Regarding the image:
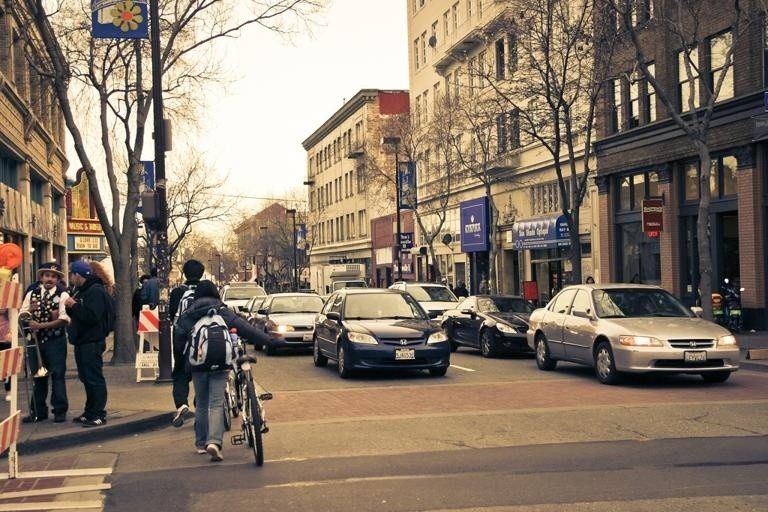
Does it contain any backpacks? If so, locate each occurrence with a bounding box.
[95,287,116,337]
[182,305,240,372]
[172,284,197,327]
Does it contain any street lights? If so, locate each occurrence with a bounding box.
[214,254,221,287]
[283,208,298,287]
[379,134,402,279]
[207,258,213,283]
[257,225,268,285]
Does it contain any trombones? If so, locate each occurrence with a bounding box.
[18,312,53,422]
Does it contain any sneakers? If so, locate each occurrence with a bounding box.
[22,412,48,423]
[197,448,208,454]
[172,404,189,428]
[206,444,223,462]
[72,411,107,427]
[54,413,66,422]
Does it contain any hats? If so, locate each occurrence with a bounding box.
[36,262,65,279]
[68,261,92,278]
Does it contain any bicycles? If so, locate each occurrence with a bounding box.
[222,328,273,469]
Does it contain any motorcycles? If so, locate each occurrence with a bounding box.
[692,284,724,327]
[721,276,747,332]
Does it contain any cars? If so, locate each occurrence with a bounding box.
[519,279,744,389]
[309,284,452,381]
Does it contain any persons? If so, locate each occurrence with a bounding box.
[448,279,469,299]
[169,259,204,428]
[140,268,158,310]
[586,277,594,284]
[132,274,158,330]
[89,262,116,295]
[64,261,116,427]
[18,263,71,423]
[173,279,281,461]
